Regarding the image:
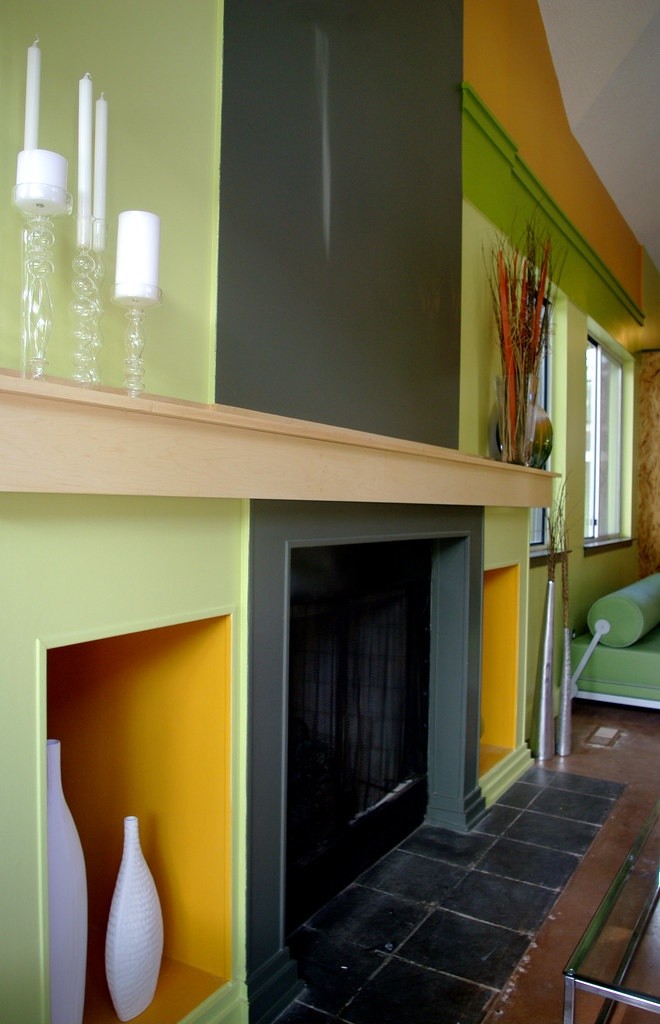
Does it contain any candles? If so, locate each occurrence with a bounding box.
[77,73,94,250]
[93,90,109,250]
[16,148,67,215]
[116,211,160,307]
[24,34,42,152]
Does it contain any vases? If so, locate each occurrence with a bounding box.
[556,627,572,756]
[488,371,553,470]
[105,815,164,1021]
[529,581,555,760]
[46,740,87,1024]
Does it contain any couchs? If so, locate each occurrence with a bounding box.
[570,573,660,709]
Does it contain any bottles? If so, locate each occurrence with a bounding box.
[105,815,163,1021]
[47,739,86,1024]
